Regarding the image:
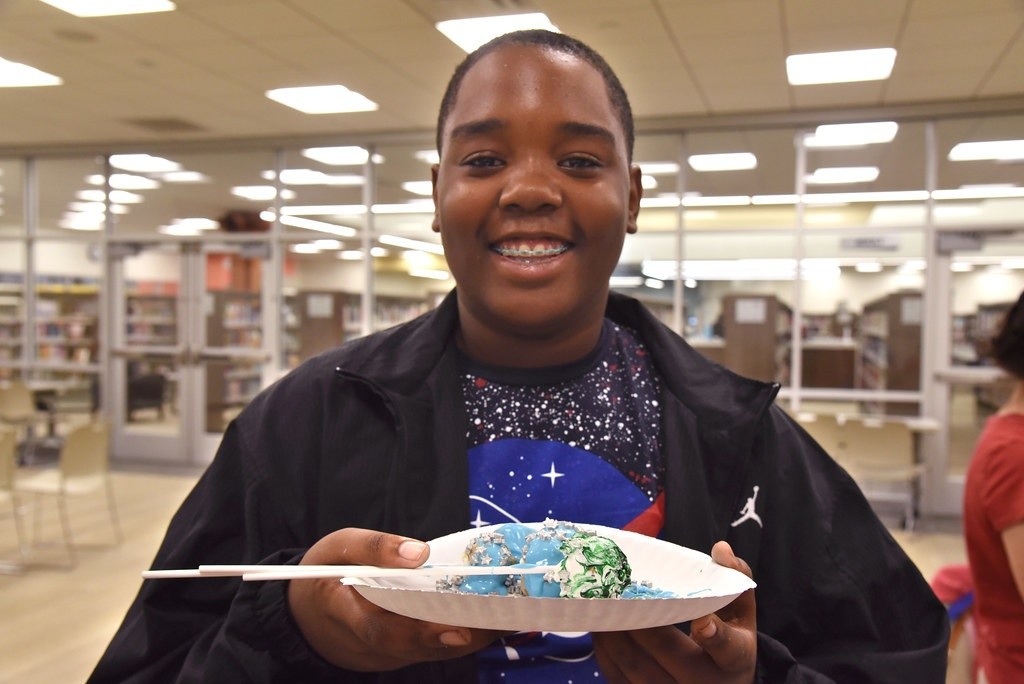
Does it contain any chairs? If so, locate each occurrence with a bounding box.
[0,377,122,575]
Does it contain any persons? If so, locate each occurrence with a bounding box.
[964,289,1023,684]
[86,29,952,684]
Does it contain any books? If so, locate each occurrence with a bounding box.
[0,297,428,432]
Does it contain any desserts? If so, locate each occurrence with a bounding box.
[452,519,634,599]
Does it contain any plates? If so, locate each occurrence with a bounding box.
[348,523,757,631]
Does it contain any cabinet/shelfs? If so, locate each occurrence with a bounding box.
[724,293,792,407]
[970,298,1015,410]
[849,289,927,417]
[0,284,427,449]
